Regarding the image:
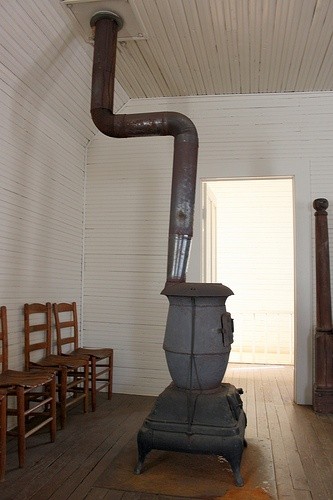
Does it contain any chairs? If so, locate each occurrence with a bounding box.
[0,302,114,482]
